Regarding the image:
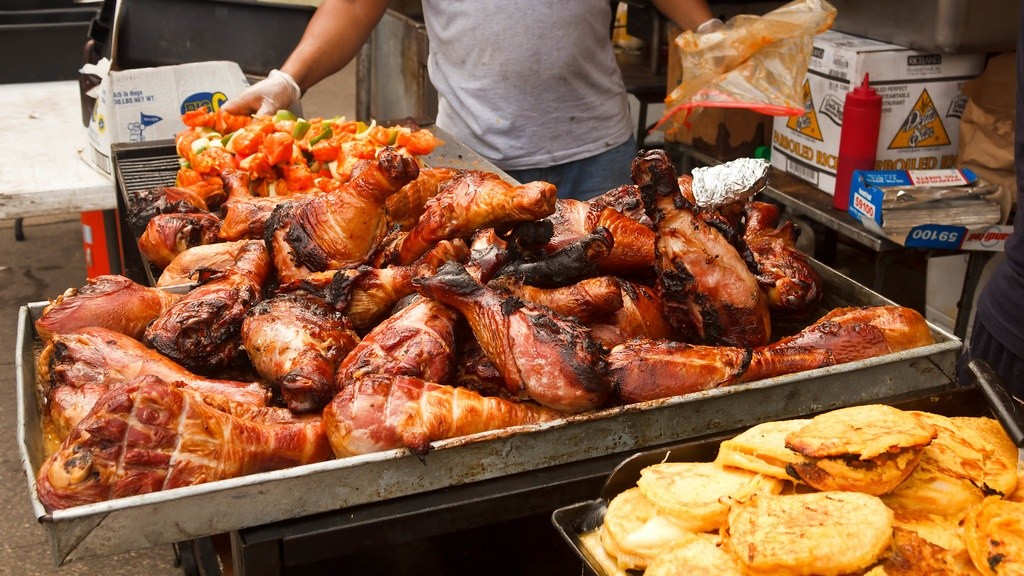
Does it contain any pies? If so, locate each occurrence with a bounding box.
[600,401,1024,576]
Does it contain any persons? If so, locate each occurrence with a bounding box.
[218,1,729,204]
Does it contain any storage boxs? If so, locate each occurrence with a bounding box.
[77,57,250,182]
[769,30,984,199]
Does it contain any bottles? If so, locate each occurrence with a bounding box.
[832,72,884,212]
